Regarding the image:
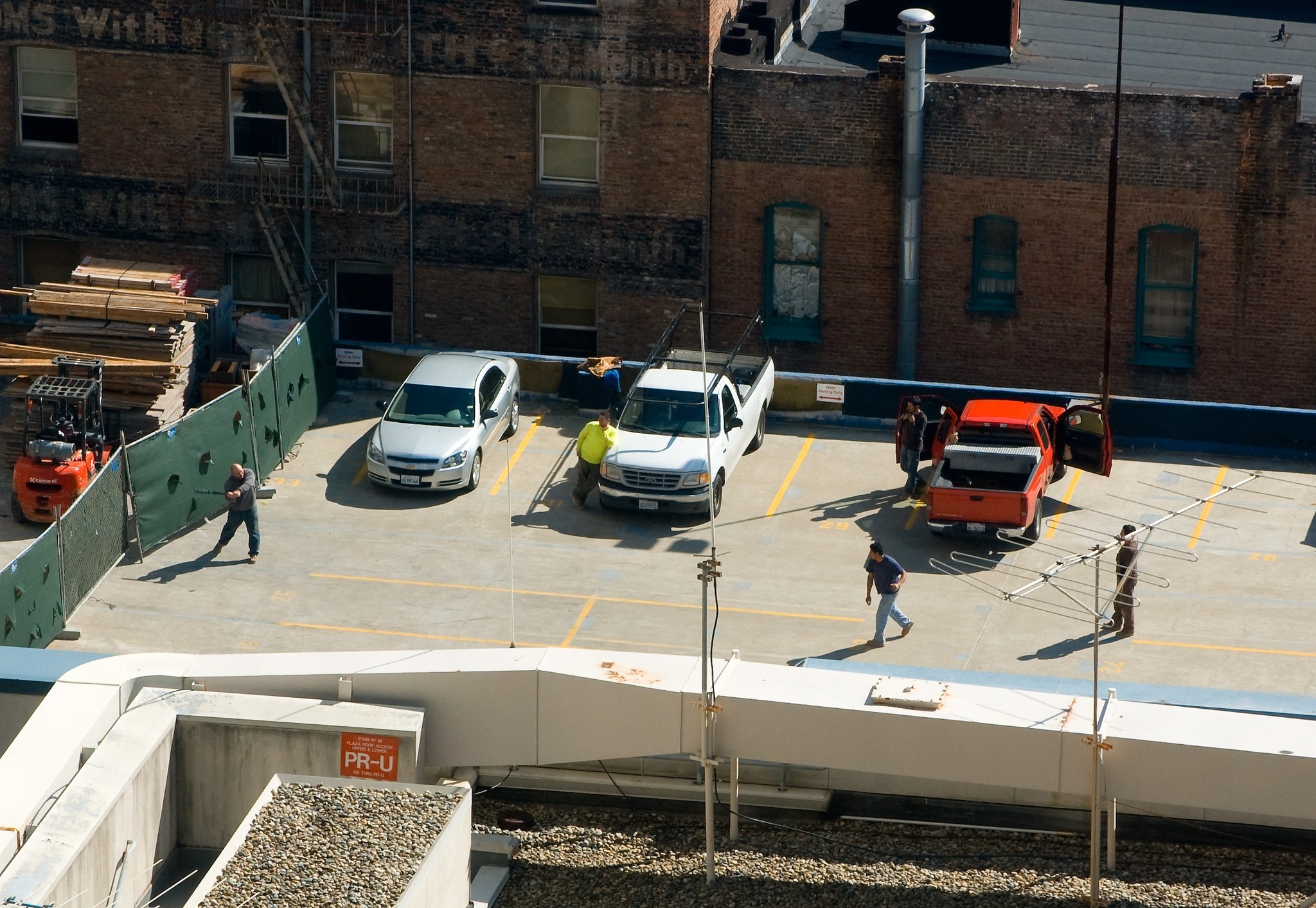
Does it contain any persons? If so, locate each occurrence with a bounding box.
[896,396,927,501]
[213,463,260,563]
[1102,524,1138,637]
[570,411,616,510]
[865,542,914,647]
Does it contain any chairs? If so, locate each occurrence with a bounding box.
[700,393,728,423]
[643,392,672,424]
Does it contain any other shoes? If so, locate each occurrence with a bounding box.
[902,620,914,636]
[915,482,927,491]
[1115,632,1134,638]
[578,504,585,509]
[570,490,582,505]
[1103,623,1121,630]
[867,640,885,647]
[900,489,913,500]
[249,554,258,563]
[213,542,223,553]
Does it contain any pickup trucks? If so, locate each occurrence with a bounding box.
[894,393,1114,543]
[597,343,775,519]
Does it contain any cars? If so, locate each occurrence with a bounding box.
[364,350,522,496]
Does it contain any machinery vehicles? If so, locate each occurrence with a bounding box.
[10,355,116,526]
